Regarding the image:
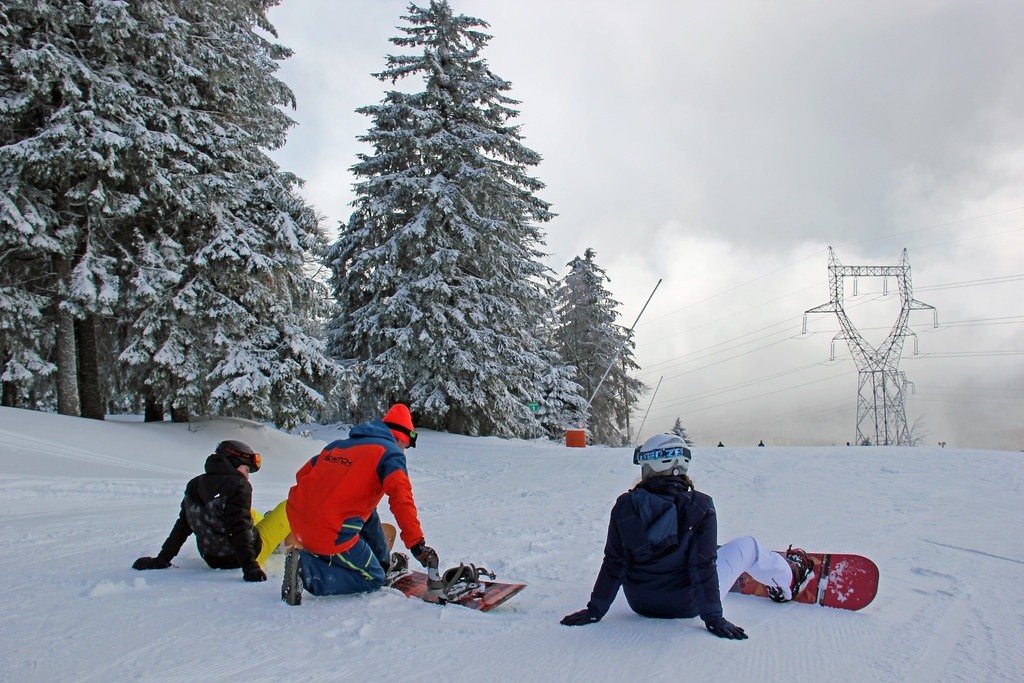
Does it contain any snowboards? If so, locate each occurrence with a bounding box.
[717,543,879,612]
[384,568,528,612]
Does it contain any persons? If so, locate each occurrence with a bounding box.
[847,442,850,446]
[282,404,439,606]
[133,440,291,582]
[758,441,764,447]
[718,442,724,447]
[560,435,810,640]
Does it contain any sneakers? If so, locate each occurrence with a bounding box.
[766,547,811,604]
[386,553,409,574]
[281,547,305,606]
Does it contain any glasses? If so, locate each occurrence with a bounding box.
[251,453,261,470]
[632,444,643,465]
[410,431,418,448]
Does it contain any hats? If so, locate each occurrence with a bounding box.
[381,403,418,449]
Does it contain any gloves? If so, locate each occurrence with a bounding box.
[131,556,170,571]
[559,609,600,627]
[241,560,267,582]
[411,539,439,568]
[704,616,749,640]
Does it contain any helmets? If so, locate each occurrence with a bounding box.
[214,440,261,473]
[633,434,692,476]
